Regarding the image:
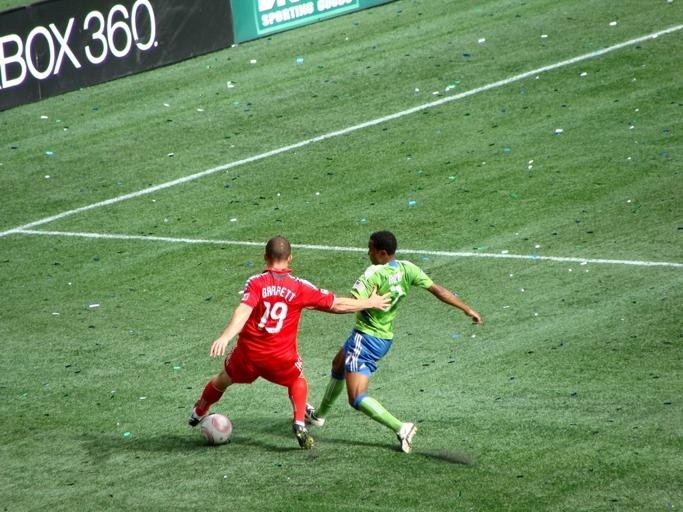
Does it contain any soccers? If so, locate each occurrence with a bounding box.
[201,413,232,445]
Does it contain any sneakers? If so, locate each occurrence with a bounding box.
[292,424,313,450]
[396,422,417,454]
[305,403,325,428]
[190,400,206,428]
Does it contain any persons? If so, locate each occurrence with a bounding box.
[303,231,482,455]
[187,235,393,448]
